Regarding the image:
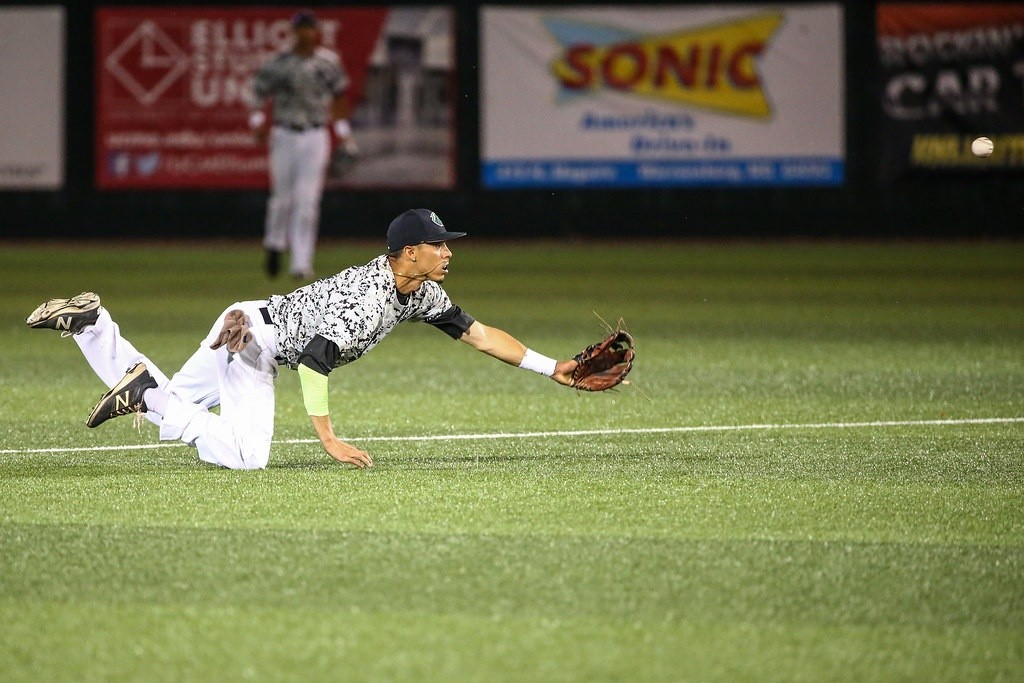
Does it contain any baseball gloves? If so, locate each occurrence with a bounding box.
[568,329,635,392]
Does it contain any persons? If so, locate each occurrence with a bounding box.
[250,15,357,280]
[26,209,635,471]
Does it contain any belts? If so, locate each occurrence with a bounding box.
[259,308,285,366]
[289,124,304,130]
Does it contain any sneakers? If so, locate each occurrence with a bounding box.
[86,362,158,433]
[25,292,101,338]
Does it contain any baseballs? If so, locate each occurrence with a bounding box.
[971,136,994,158]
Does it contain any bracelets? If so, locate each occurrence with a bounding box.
[519,348,557,377]
[334,119,350,136]
[250,111,261,127]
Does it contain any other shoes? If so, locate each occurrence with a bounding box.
[264,251,281,278]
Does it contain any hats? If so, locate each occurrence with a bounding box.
[386,209,467,251]
[294,11,317,27]
